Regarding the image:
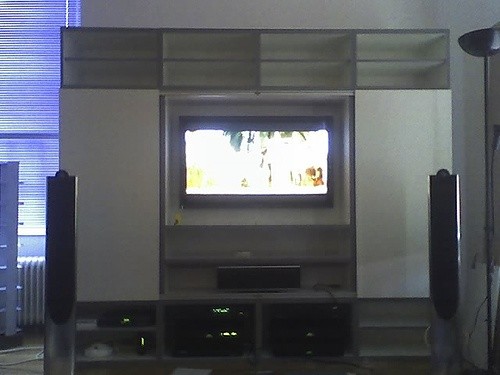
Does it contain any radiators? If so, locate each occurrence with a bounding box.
[18,255,46,328]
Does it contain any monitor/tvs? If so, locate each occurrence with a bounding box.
[178,114,336,207]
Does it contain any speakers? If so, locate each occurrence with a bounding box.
[46,170,76,372]
[426,168,461,371]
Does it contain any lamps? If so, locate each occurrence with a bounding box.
[457,26,499,374]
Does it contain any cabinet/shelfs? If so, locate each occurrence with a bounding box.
[61,28,450,90]
[75,297,435,375]
